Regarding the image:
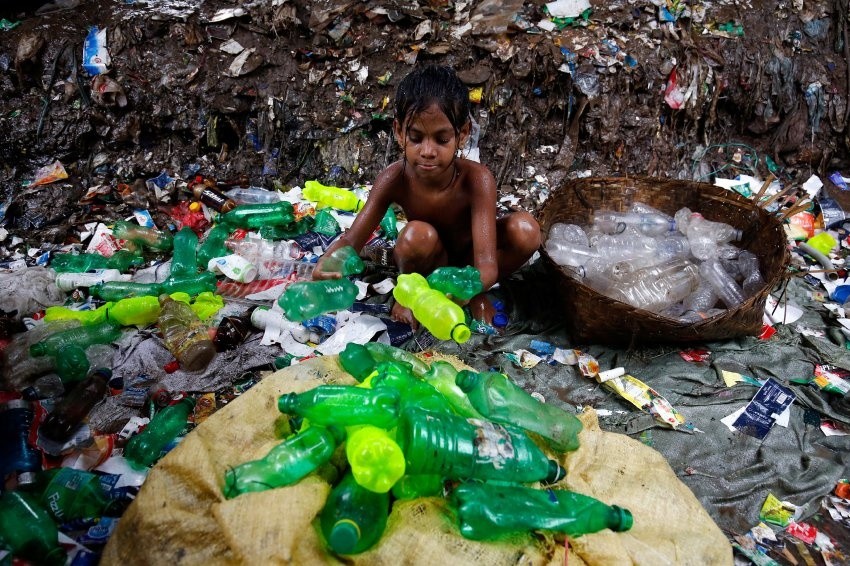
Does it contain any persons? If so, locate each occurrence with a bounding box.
[312,66,540,334]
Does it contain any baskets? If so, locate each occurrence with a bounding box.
[538,175,788,348]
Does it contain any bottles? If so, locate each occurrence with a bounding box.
[0,135,765,566]
